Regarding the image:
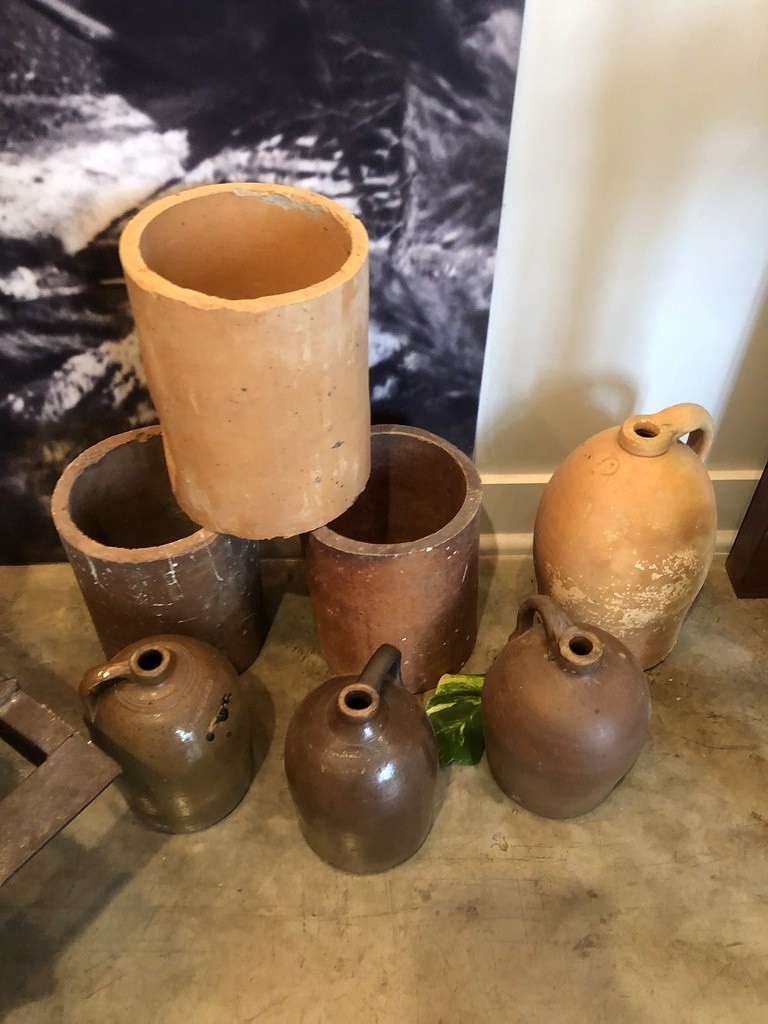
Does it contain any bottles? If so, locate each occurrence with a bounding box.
[284,642,438,874]
[479,596,652,817]
[80,640,254,836]
[530,403,715,671]
